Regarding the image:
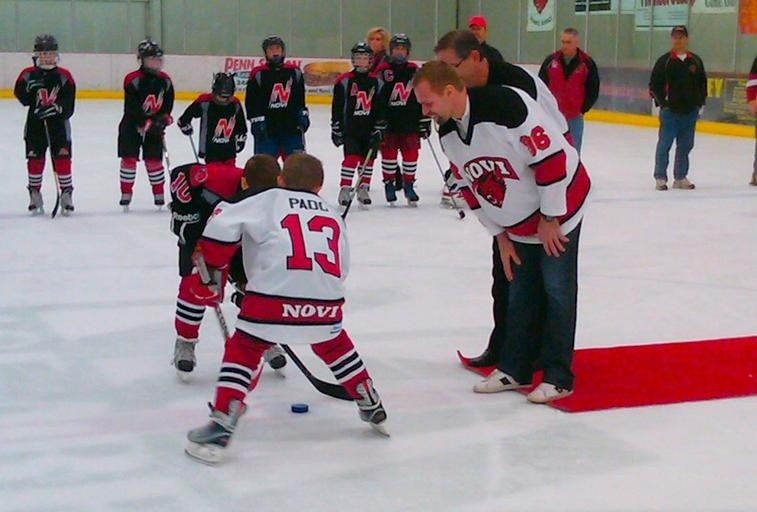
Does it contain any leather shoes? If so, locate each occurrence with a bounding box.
[468,348,498,367]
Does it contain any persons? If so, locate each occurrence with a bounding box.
[540,28,600,153]
[166,149,388,448]
[435,29,574,367]
[176,69,248,166]
[244,32,311,164]
[331,28,431,210]
[746,55,757,185]
[13,33,79,214]
[115,38,176,205]
[646,23,709,192]
[411,59,591,402]
[440,16,504,209]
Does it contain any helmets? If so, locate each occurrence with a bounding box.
[33,34,59,52]
[352,42,376,73]
[386,34,409,61]
[259,35,285,54]
[213,72,235,98]
[137,39,161,58]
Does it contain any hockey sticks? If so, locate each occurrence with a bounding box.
[193,249,264,393]
[281,344,358,401]
[426,134,466,220]
[42,120,60,216]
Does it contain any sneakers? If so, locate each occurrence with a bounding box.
[475,367,532,393]
[654,176,694,189]
[25,188,43,211]
[188,399,246,446]
[61,187,75,210]
[339,181,419,205]
[155,194,166,205]
[526,383,573,403]
[264,346,286,368]
[171,337,197,371]
[120,193,132,206]
[352,380,386,423]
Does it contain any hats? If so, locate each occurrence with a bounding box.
[469,15,487,29]
[672,24,688,34]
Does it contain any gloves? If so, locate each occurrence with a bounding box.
[188,265,228,302]
[418,116,433,139]
[177,118,194,134]
[252,117,265,136]
[168,202,204,246]
[39,102,60,123]
[234,134,246,152]
[371,122,386,143]
[330,121,342,145]
[301,108,312,130]
